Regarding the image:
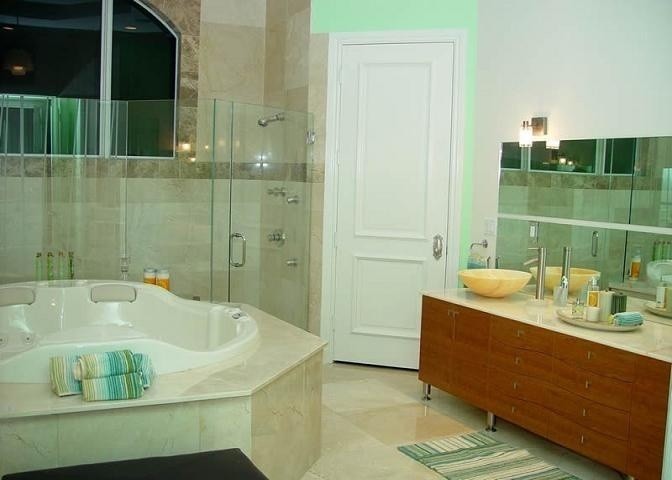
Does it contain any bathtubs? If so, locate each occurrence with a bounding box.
[0,278,259,382]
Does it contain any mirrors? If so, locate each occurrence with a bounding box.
[495,137,672,304]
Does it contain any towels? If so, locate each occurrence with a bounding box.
[46,350,156,401]
[611,310,645,327]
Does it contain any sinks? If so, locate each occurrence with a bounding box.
[458,268,532,298]
[530,265,600,291]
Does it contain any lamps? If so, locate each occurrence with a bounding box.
[520,116,548,147]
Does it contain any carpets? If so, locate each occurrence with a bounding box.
[397,431,583,480]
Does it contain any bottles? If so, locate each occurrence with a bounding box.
[144,268,169,289]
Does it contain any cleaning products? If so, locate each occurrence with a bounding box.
[631,249,642,282]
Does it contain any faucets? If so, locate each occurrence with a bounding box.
[562,248,571,292]
[524,246,547,301]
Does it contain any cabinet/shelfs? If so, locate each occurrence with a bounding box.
[418,296,668,478]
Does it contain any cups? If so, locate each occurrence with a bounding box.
[586,306,601,320]
